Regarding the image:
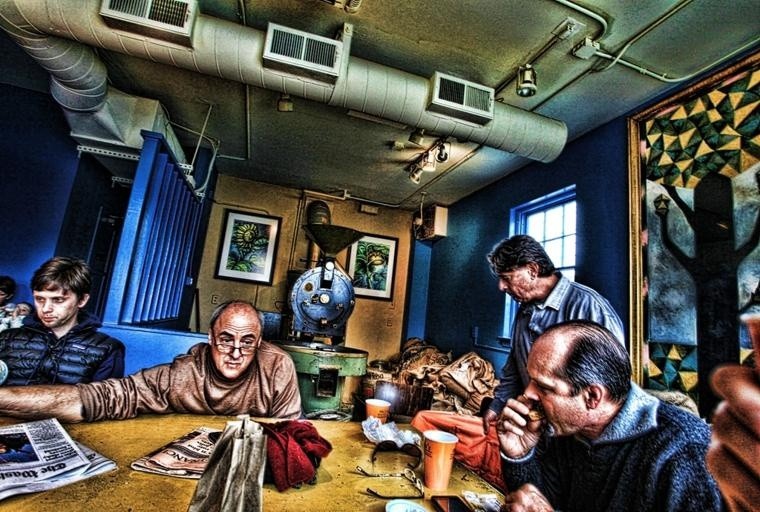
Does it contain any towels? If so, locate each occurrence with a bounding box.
[260,419,334,490]
[184,414,265,512]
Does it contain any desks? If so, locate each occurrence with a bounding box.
[1,409,513,512]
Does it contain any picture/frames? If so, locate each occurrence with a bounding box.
[347,232,400,301]
[626,53,760,424]
[213,208,282,287]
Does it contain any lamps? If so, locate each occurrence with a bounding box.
[279,90,299,111]
[405,132,451,192]
[516,60,538,101]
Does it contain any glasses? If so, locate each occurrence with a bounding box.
[373,440,423,469]
[356,466,426,498]
[212,328,261,356]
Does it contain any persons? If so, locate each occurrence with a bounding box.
[0,275,16,318]
[0,302,35,332]
[484,236,626,458]
[495,318,729,512]
[0,256,126,385]
[0,299,303,424]
[706,318,760,512]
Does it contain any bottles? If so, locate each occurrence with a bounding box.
[4,304,18,313]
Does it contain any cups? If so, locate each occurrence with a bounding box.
[365,397,392,425]
[421,430,460,492]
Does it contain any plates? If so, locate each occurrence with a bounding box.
[384,497,428,512]
